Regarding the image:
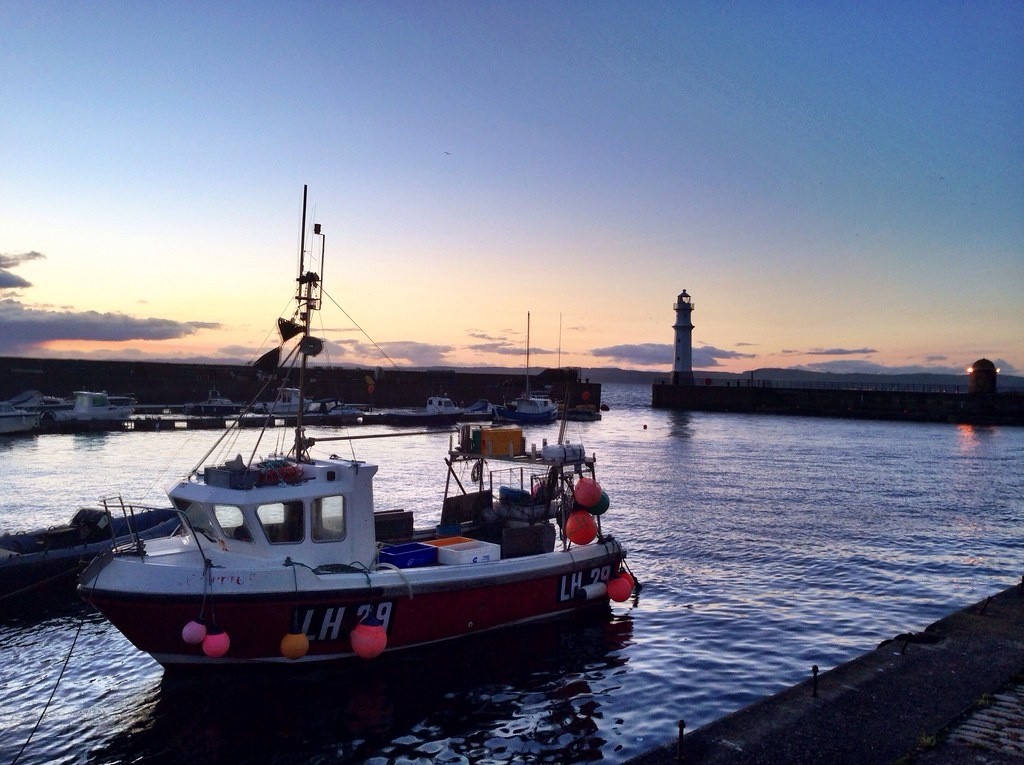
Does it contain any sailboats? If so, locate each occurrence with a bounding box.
[490,309,559,424]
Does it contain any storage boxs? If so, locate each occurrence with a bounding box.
[473,427,524,456]
[204,467,260,490]
[425,536,501,565]
[33,527,80,549]
[498,502,548,518]
[378,542,438,570]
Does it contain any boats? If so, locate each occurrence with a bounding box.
[385,394,466,426]
[183,385,255,416]
[74,179,629,671]
[0,504,189,605]
[0,386,139,436]
[253,377,314,416]
